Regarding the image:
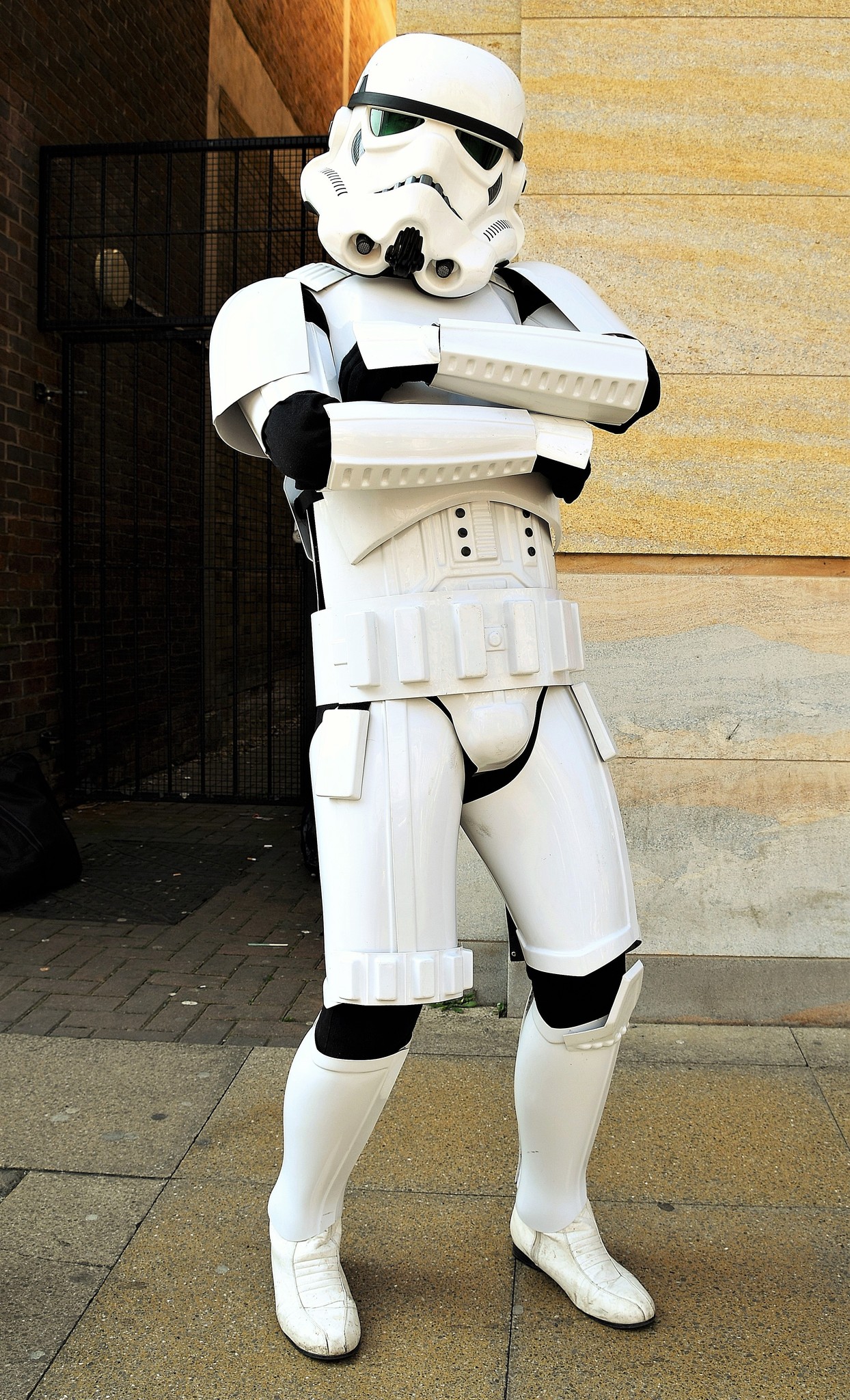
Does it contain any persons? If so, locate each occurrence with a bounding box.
[203,33,660,1361]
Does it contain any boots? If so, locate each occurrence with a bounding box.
[509,960,656,1331]
[266,1013,412,1362]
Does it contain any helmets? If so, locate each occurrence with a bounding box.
[302,33,530,297]
